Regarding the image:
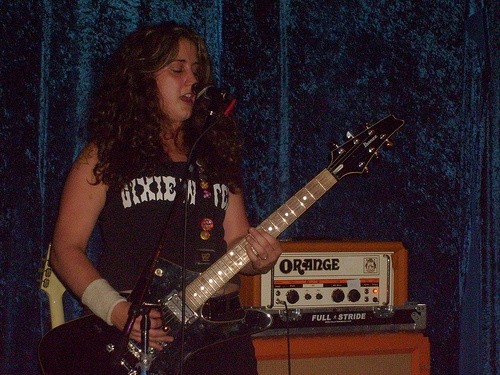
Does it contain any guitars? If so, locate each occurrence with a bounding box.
[36,113,406,375]
[35,241,68,331]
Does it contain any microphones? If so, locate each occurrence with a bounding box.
[191,82,234,102]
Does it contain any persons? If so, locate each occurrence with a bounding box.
[49,20,282,375]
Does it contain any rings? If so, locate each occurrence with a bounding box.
[261,254,268,260]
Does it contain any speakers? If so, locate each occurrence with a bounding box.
[252,330,432,375]
